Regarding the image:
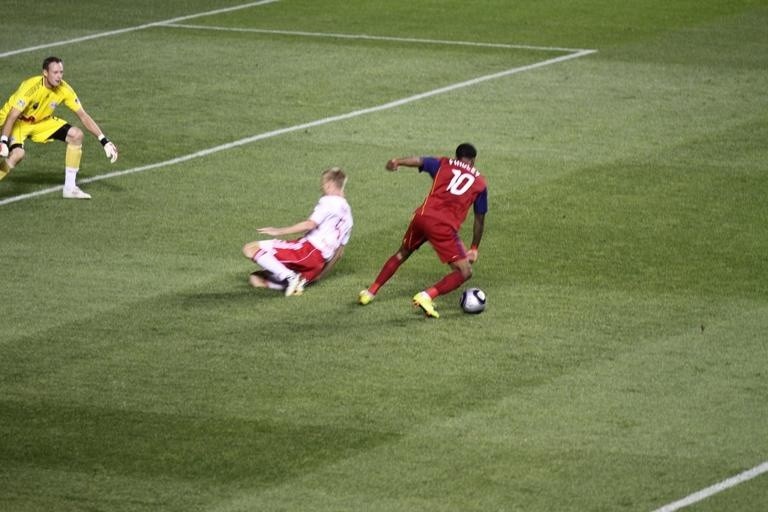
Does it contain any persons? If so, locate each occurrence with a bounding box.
[356,139,491,321]
[0,52,120,202]
[238,164,355,298]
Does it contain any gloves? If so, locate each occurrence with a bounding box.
[103,142,118,164]
[0,142,9,157]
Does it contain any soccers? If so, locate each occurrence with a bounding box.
[460,288,486,312]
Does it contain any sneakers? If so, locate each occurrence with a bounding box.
[63,186,91,199]
[284,272,309,297]
[358,288,375,305]
[412,292,440,319]
